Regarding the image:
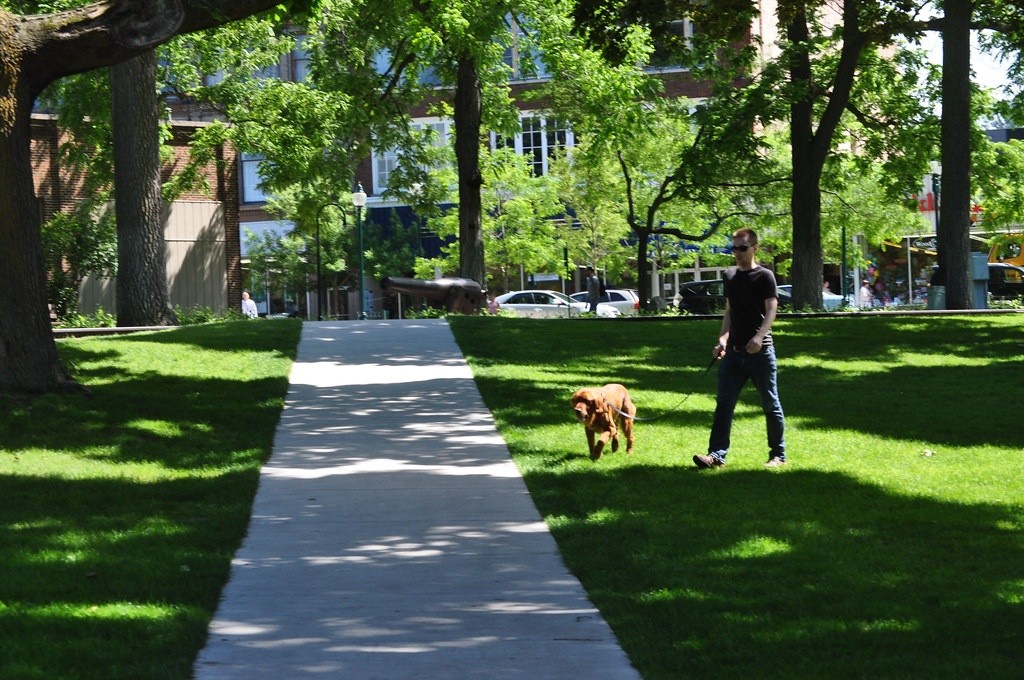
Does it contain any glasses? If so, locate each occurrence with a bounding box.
[731,244,753,252]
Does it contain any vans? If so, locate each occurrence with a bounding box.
[985,233,1024,285]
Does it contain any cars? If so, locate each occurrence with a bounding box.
[674,278,792,316]
[553,288,649,316]
[491,290,623,320]
[930,262,1024,307]
[776,284,851,313]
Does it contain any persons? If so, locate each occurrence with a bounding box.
[487,295,499,314]
[241,292,258,317]
[693,228,786,469]
[584,266,600,314]
[860,280,873,307]
[823,281,835,295]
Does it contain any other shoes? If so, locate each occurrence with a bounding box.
[764,457,784,467]
[693,455,716,468]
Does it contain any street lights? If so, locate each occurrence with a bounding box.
[315,203,348,323]
[350,180,368,319]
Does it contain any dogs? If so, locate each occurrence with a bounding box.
[572,384,636,463]
